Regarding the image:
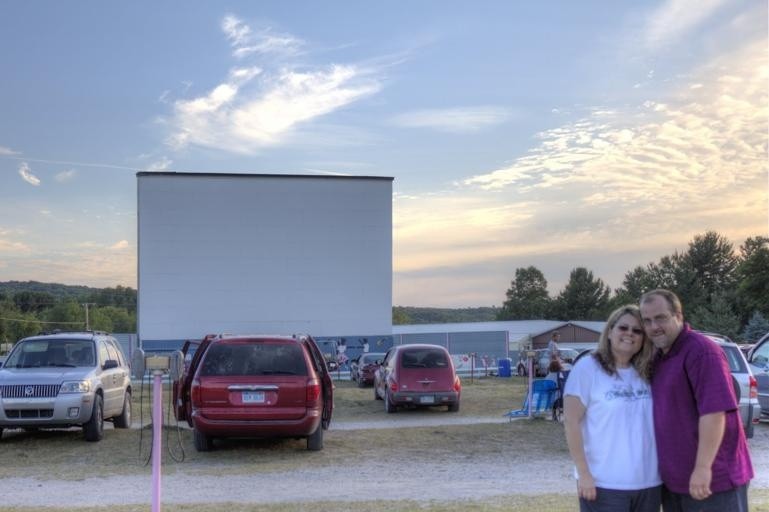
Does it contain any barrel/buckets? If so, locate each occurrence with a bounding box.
[498,359,511,378]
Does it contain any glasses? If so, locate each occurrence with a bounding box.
[616,325,642,334]
[643,314,676,323]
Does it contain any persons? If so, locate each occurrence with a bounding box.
[358,337,370,352]
[563,301,665,511]
[637,289,755,511]
[548,330,563,372]
[335,338,350,371]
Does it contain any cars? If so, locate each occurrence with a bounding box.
[0,331,132,443]
[517,348,580,377]
[173,335,334,452]
[351,353,388,387]
[373,344,461,413]
[696,330,769,440]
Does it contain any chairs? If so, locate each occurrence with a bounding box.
[557,369,571,422]
[505,380,556,422]
